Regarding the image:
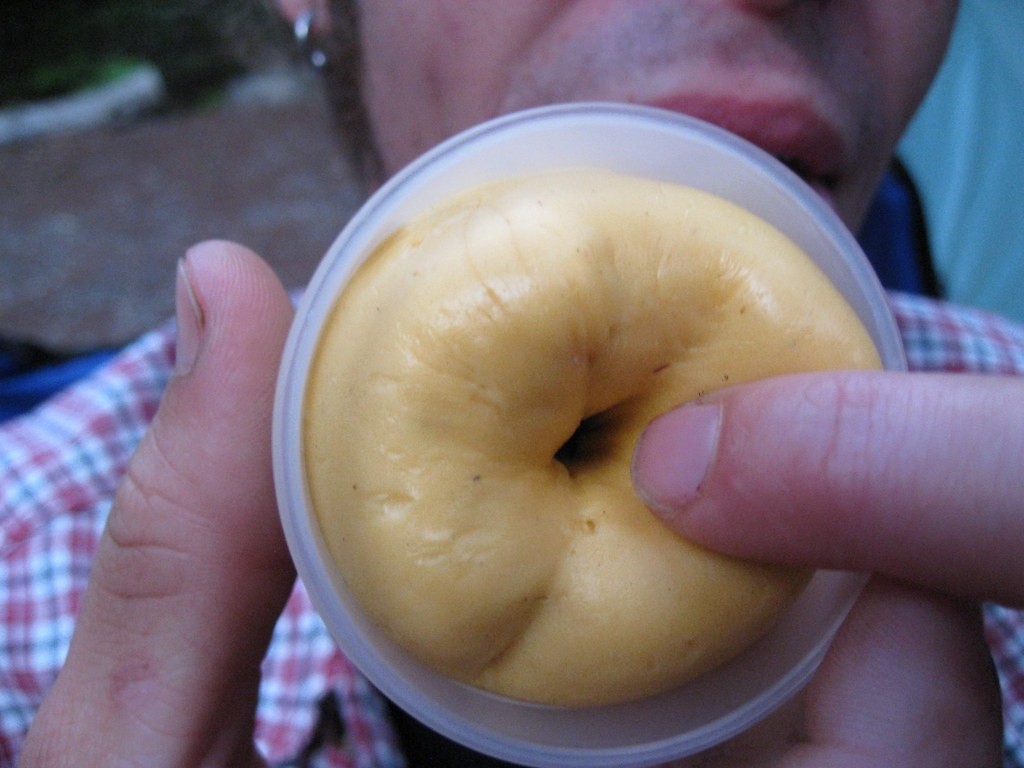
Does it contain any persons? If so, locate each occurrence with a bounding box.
[0,0,1024,768]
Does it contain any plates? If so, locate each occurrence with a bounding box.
[269,101,910,768]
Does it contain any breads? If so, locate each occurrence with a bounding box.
[293,163,889,716]
